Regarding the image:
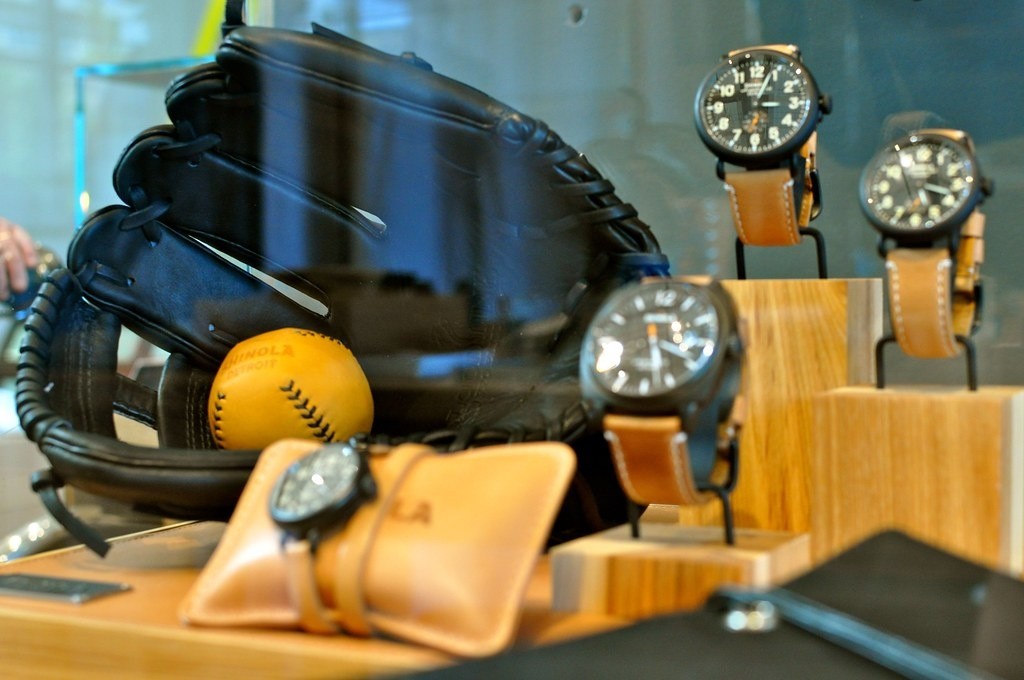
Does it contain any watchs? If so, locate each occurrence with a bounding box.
[581,277,752,504]
[695,44,832,245]
[860,128,993,358]
[270,435,397,630]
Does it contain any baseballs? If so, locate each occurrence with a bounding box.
[208,326,379,447]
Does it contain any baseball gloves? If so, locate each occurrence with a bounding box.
[10,26,708,560]
